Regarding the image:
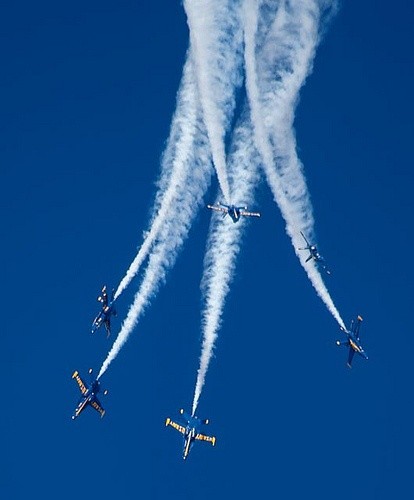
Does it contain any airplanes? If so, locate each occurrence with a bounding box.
[207,201,261,222]
[71,367,108,421]
[165,408,215,462]
[90,285,117,337]
[336,315,369,368]
[298,231,330,274]
